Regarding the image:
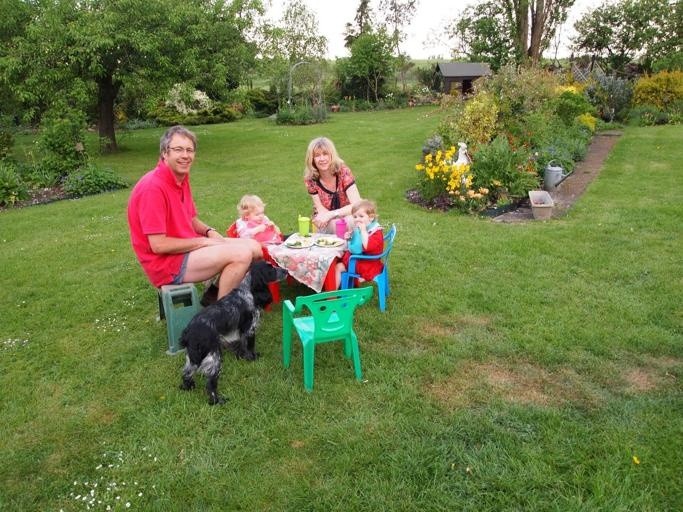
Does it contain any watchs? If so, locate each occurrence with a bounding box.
[206,228,215,236]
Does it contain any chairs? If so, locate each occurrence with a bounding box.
[225,221,293,307]
[277,284,376,393]
[339,222,398,313]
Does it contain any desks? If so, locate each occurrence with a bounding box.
[263,233,342,312]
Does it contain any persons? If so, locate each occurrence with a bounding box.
[331,202,385,300]
[305,138,360,232]
[236,196,281,282]
[127,126,260,316]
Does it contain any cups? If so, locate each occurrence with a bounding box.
[335,219,348,239]
[298,217,310,237]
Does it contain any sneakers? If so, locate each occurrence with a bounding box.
[200,291,218,306]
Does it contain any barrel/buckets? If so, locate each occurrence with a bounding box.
[347,226,362,255]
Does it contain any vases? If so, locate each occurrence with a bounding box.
[526,188,555,223]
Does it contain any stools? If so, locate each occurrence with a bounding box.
[154,282,201,356]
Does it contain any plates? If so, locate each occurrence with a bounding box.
[285,239,314,249]
[314,236,345,248]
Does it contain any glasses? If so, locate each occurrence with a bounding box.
[167,146,195,153]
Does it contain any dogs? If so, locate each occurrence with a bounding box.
[166,258,288,407]
